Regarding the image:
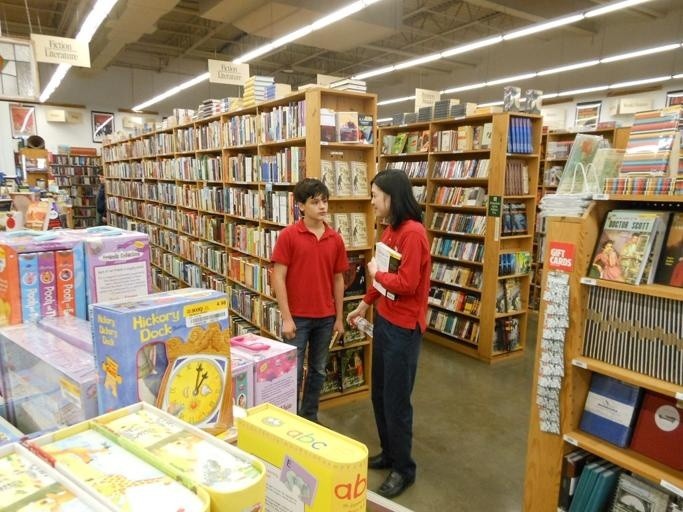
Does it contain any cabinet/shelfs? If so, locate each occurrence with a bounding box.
[98,82,383,412]
[370,109,544,367]
[528,121,628,312]
[17,140,112,233]
[521,191,683,512]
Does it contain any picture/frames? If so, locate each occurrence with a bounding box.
[90,111,114,144]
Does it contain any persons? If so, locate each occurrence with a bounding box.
[348,352,363,387]
[619,234,643,283]
[239,395,244,407]
[345,171,431,498]
[324,356,338,380]
[592,240,623,283]
[270,178,350,422]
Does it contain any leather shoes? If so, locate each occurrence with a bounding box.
[368,452,393,470]
[377,462,417,498]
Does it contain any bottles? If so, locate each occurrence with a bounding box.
[350,315,374,338]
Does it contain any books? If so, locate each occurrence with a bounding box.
[103,179,159,203]
[496,278,526,316]
[66,187,95,197]
[562,446,683,512]
[107,212,161,245]
[504,119,540,154]
[377,99,497,127]
[241,77,293,107]
[96,169,107,225]
[494,316,522,352]
[163,275,179,290]
[432,238,483,260]
[151,267,162,287]
[68,208,96,217]
[54,177,94,186]
[162,253,177,273]
[431,265,483,288]
[432,152,489,177]
[51,155,103,166]
[601,111,683,194]
[578,373,639,447]
[321,160,337,197]
[586,210,672,284]
[103,157,158,179]
[3,190,66,229]
[431,307,482,344]
[359,114,374,145]
[432,116,491,153]
[431,286,482,314]
[503,159,533,197]
[195,95,238,119]
[558,133,605,191]
[156,107,190,129]
[335,213,351,248]
[382,155,430,176]
[323,213,332,231]
[581,286,683,386]
[432,211,485,233]
[500,202,529,236]
[179,114,227,287]
[160,230,178,249]
[338,252,366,296]
[350,161,369,197]
[268,92,306,224]
[160,181,178,203]
[658,212,683,289]
[340,350,364,390]
[309,352,341,397]
[334,160,352,196]
[152,246,161,265]
[50,167,96,177]
[342,301,366,343]
[156,130,174,156]
[160,156,176,178]
[66,218,95,227]
[336,112,358,142]
[69,197,95,208]
[352,212,368,249]
[372,241,403,302]
[379,125,432,156]
[529,133,573,311]
[106,194,160,224]
[162,207,177,226]
[320,109,335,139]
[223,108,265,334]
[103,136,156,162]
[432,183,488,206]
[330,80,369,92]
[498,252,531,275]
[629,385,682,471]
